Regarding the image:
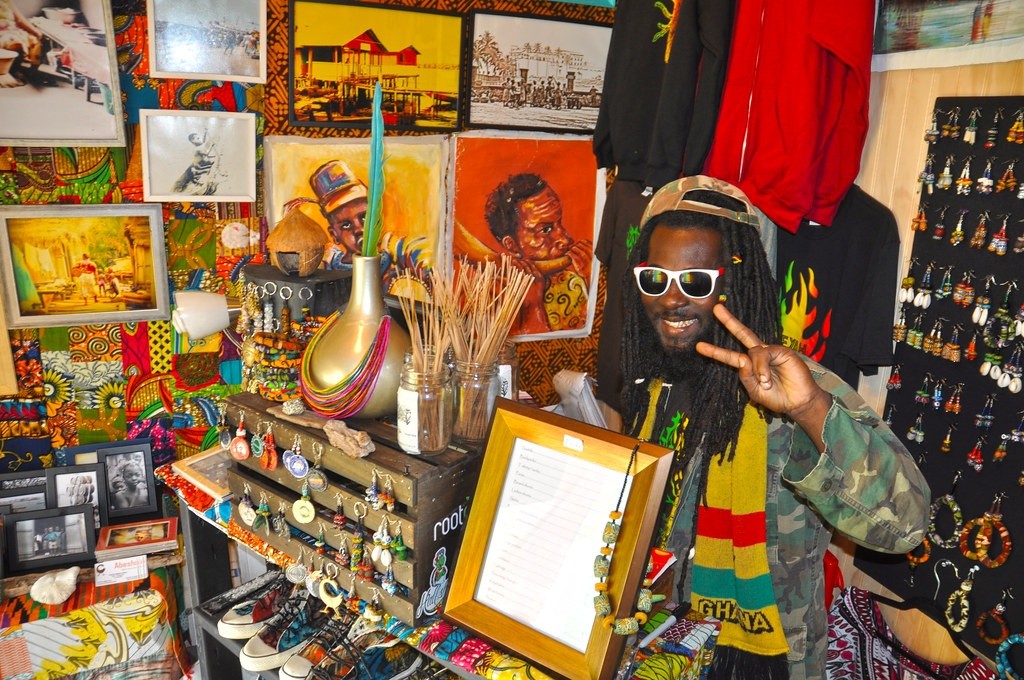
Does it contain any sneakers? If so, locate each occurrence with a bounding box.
[218,572,294,639]
[305,626,463,680]
[279,607,356,680]
[239,586,326,672]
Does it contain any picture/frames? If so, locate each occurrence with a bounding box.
[438,394,679,680]
[0,443,158,579]
[0,0,616,350]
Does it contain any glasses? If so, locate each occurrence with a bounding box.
[633,262,725,300]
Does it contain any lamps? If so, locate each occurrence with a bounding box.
[171,291,243,342]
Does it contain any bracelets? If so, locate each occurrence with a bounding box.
[254,317,327,401]
[908,495,1024,680]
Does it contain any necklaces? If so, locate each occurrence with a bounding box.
[300,299,391,418]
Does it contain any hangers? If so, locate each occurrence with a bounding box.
[837,556,1005,680]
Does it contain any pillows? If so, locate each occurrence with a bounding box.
[0,566,194,680]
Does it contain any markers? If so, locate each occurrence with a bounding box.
[637,600,692,649]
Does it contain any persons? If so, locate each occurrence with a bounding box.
[0,0,42,75]
[110,460,149,510]
[34,523,67,556]
[592,172,933,680]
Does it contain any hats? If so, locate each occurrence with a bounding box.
[639,175,762,238]
